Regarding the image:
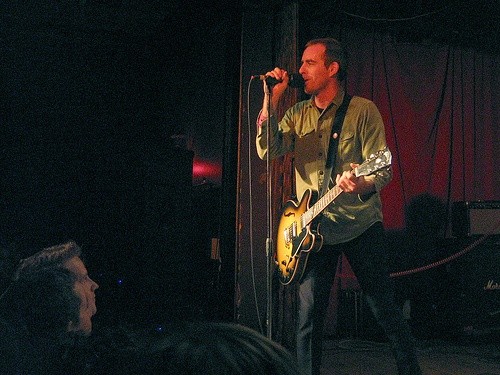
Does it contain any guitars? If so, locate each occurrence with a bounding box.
[275,145,393,286]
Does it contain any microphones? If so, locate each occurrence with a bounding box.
[254,72,304,88]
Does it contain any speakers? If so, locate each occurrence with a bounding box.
[453,243,500,340]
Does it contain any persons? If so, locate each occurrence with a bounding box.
[1,267,98,375]
[118,320,303,375]
[14,242,97,323]
[255,35,424,375]
